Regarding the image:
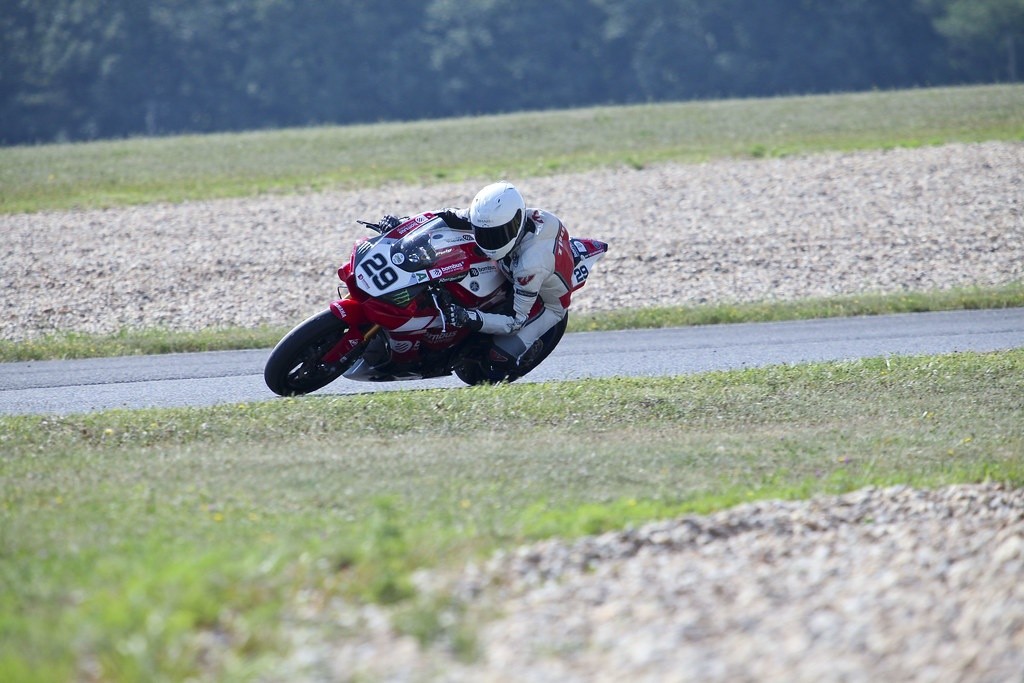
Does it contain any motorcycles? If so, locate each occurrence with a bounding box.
[263,211,609,396]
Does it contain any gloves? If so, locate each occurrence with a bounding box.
[379,215,402,231]
[441,303,483,330]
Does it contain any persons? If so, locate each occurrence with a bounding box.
[379,181,574,385]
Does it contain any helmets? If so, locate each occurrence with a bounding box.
[470,181,525,260]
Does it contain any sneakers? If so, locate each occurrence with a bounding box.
[516,340,544,371]
